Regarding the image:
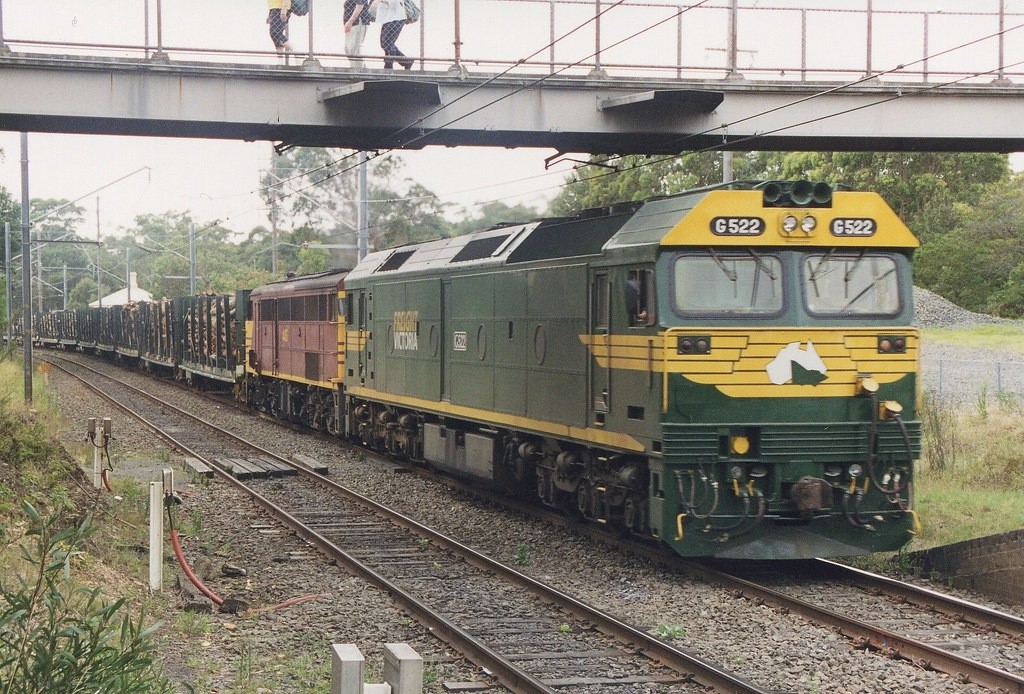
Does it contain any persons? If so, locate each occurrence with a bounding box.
[343,0,370,68]
[266,0,305,65]
[376,0,414,70]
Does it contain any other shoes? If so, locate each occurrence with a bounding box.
[405,59,415,69]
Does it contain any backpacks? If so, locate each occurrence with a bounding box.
[290,0,308,16]
[404,0,420,24]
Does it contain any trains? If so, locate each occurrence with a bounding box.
[11,180,922,560]
